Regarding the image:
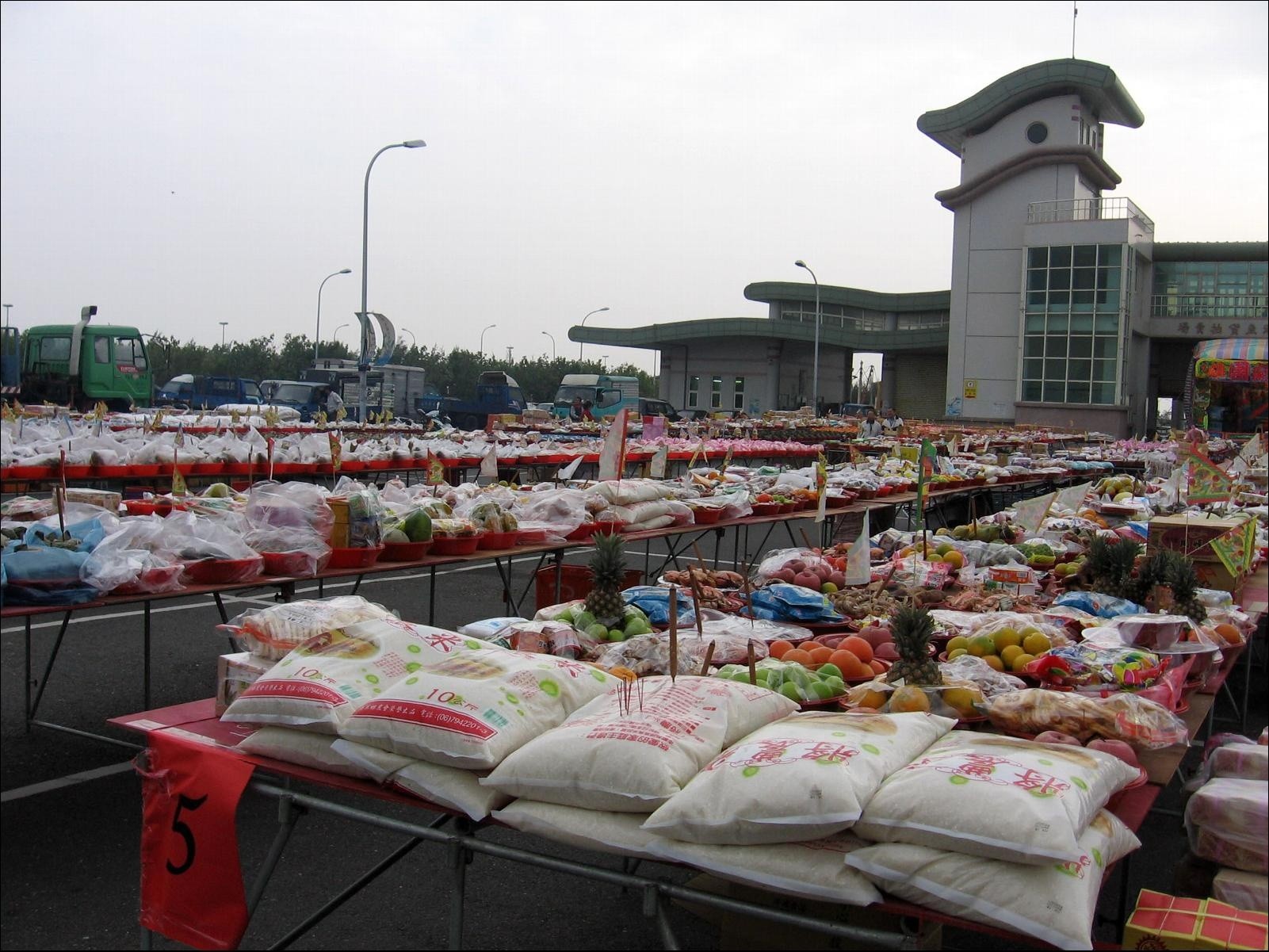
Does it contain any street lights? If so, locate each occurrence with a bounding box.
[314,268,353,360]
[357,138,428,425]
[505,346,514,365]
[542,331,556,364]
[402,328,415,348]
[602,355,610,370]
[480,324,496,359]
[795,259,820,413]
[219,322,229,346]
[580,307,610,361]
[333,324,350,345]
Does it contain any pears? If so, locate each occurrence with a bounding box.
[552,604,654,642]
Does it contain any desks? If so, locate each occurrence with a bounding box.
[0,458,1269,952]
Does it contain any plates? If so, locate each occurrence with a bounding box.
[579,539,1258,791]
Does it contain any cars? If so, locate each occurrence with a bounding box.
[714,409,751,420]
[677,410,711,421]
[534,402,555,415]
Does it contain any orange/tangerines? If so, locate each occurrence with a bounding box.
[770,637,885,680]
[946,627,1050,672]
[1096,477,1141,496]
[1179,624,1241,645]
[898,542,963,569]
[844,677,983,718]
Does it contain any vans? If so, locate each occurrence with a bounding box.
[550,374,640,421]
[844,403,874,419]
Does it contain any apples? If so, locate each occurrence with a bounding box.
[774,544,884,593]
[713,658,844,700]
[1078,509,1108,528]
[1032,729,1137,768]
[1055,555,1086,575]
[823,626,900,660]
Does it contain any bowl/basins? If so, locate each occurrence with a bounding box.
[1,425,1152,603]
[1113,612,1190,648]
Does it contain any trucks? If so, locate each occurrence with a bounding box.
[151,370,387,410]
[0,306,154,413]
[414,371,529,432]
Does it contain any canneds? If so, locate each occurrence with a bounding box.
[490,625,610,665]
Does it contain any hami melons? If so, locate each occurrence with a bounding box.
[382,529,409,542]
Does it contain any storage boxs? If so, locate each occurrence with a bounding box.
[1146,516,1236,562]
[216,651,277,718]
[988,566,1028,582]
[998,582,1035,596]
[533,564,644,609]
[488,409,547,431]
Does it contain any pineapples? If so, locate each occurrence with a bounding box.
[1084,531,1208,626]
[579,531,625,631]
[885,604,943,689]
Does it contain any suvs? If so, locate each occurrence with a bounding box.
[638,398,684,422]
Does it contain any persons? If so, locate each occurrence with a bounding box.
[313,385,344,421]
[569,396,594,422]
[881,407,904,431]
[857,409,881,439]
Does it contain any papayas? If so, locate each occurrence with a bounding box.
[935,522,1007,544]
[404,509,432,542]
